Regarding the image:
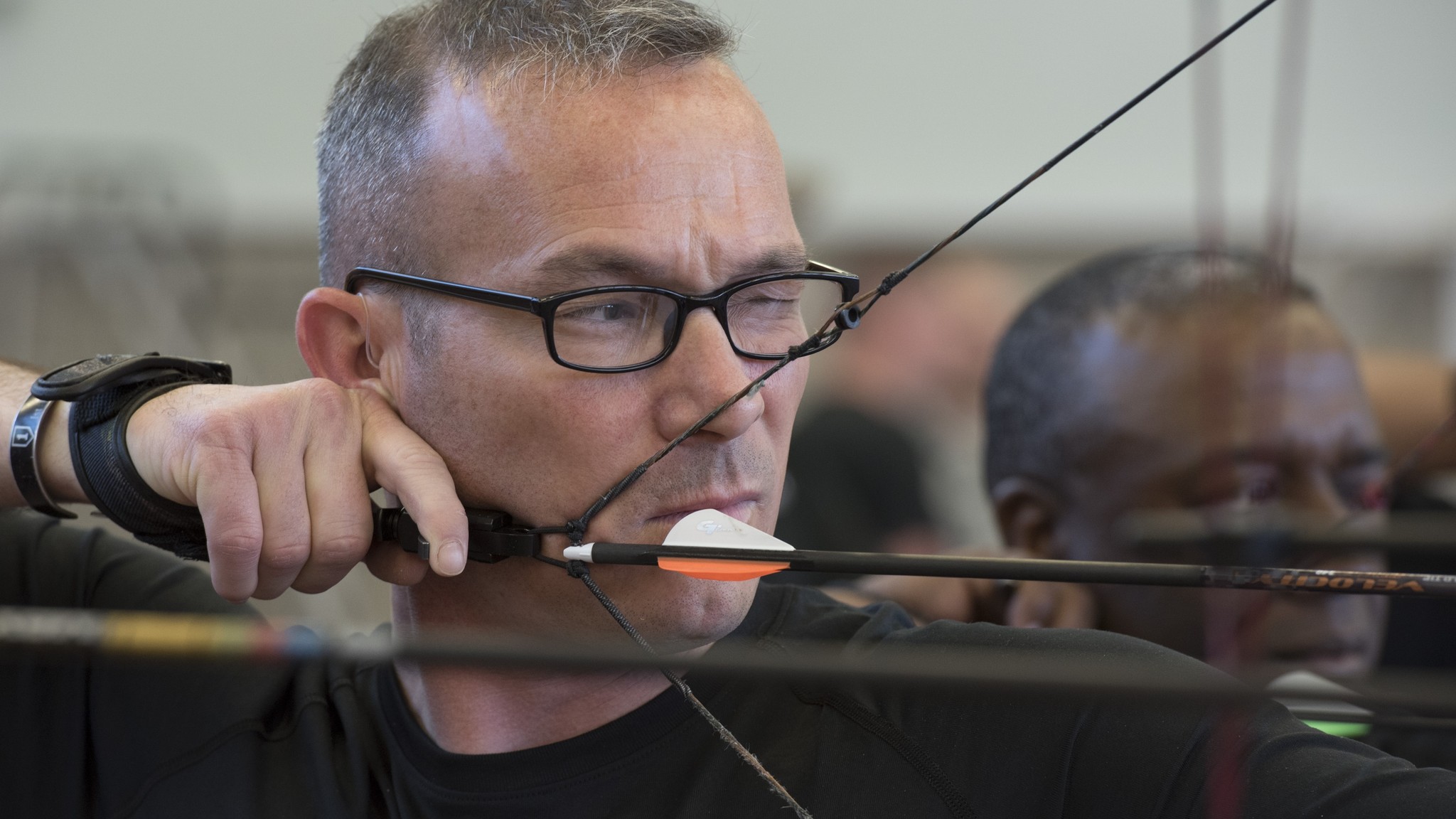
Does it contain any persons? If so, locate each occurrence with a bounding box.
[816,242,1392,737]
[0,2,1452,818]
[782,395,939,594]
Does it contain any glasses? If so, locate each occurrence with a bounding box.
[343,258,862,374]
